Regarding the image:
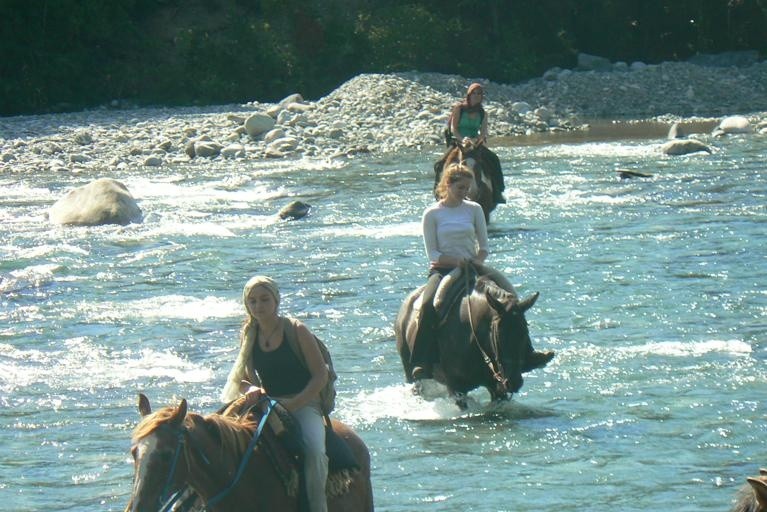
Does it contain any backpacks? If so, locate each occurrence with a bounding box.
[286,318,337,416]
[444,102,484,148]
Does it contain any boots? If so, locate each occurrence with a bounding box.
[494,191,506,204]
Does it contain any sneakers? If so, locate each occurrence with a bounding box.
[412,367,423,379]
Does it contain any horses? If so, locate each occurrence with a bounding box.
[119,389,374,512]
[441,133,497,225]
[393,273,540,413]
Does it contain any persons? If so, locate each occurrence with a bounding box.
[411,164,554,380]
[434,83,506,203]
[221,274,336,512]
